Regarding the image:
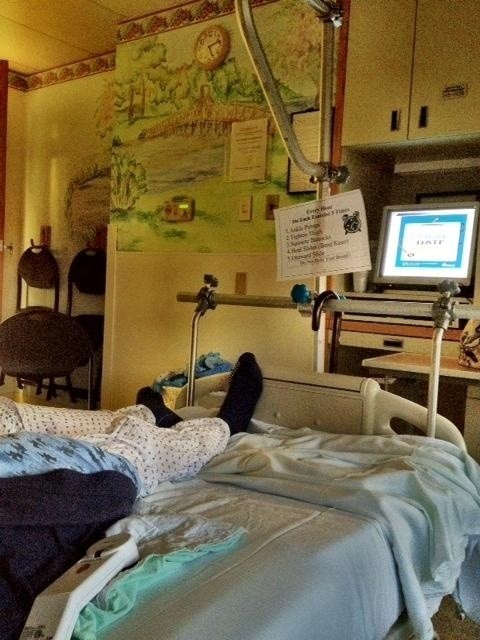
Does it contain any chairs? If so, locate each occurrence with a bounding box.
[0,245,107,404]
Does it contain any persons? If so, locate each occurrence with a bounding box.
[0,350,263,638]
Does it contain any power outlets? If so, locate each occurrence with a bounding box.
[265,194,279,220]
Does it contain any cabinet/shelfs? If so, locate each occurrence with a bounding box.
[340,0,480,153]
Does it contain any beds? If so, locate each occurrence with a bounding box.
[71,367,470,640]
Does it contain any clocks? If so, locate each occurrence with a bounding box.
[193,25,230,71]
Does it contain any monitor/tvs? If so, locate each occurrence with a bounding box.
[371,201,480,297]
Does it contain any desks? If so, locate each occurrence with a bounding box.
[326,290,480,387]
